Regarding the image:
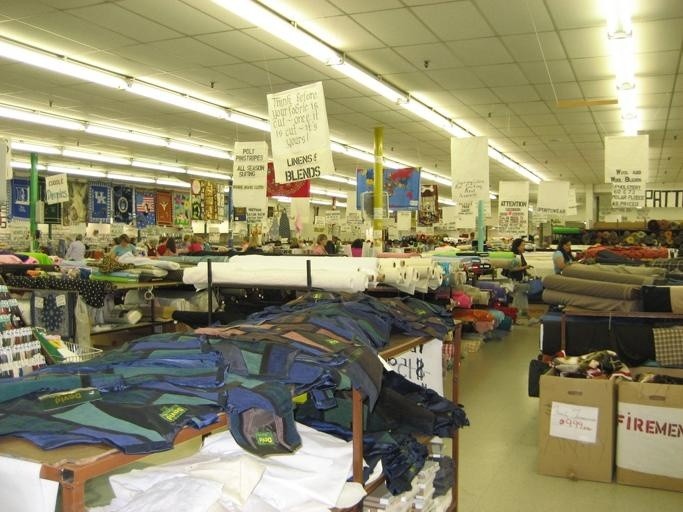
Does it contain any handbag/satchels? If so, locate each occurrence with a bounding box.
[501,269,523,281]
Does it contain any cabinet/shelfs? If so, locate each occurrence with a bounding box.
[0,280,462,511]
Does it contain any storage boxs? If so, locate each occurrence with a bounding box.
[536,360,683,492]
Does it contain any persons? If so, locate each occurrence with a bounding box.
[508,238,540,326]
[109,234,209,260]
[62,233,86,261]
[241,236,249,251]
[467,232,475,245]
[551,238,574,275]
[290,238,299,248]
[313,234,328,255]
[351,238,362,257]
[90,230,99,243]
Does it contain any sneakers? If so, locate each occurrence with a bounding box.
[513,319,523,325]
[528,317,539,326]
